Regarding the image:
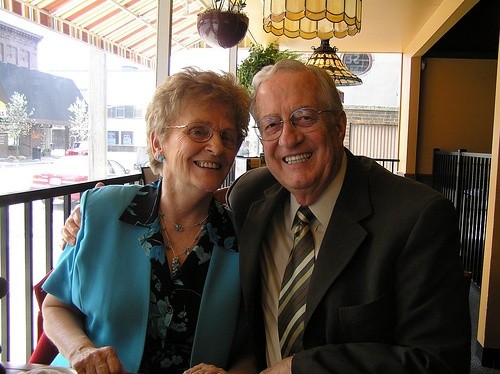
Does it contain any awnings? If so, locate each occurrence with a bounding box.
[0,0,256,73]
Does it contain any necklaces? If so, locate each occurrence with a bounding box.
[156,210,210,280]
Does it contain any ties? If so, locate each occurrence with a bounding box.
[275,206,315,360]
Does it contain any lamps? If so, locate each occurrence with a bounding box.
[305,40,363,86]
[263,0,363,40]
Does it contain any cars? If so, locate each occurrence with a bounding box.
[107,143,149,176]
[66,141,88,156]
[30,149,132,205]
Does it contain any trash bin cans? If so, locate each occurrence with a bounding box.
[32,148,41,160]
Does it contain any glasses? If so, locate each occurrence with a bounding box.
[252,106,335,142]
[162,121,247,150]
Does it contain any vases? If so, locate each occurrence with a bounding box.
[197,13,249,49]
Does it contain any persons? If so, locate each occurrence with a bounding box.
[41,65,259,374]
[60,59,474,374]
